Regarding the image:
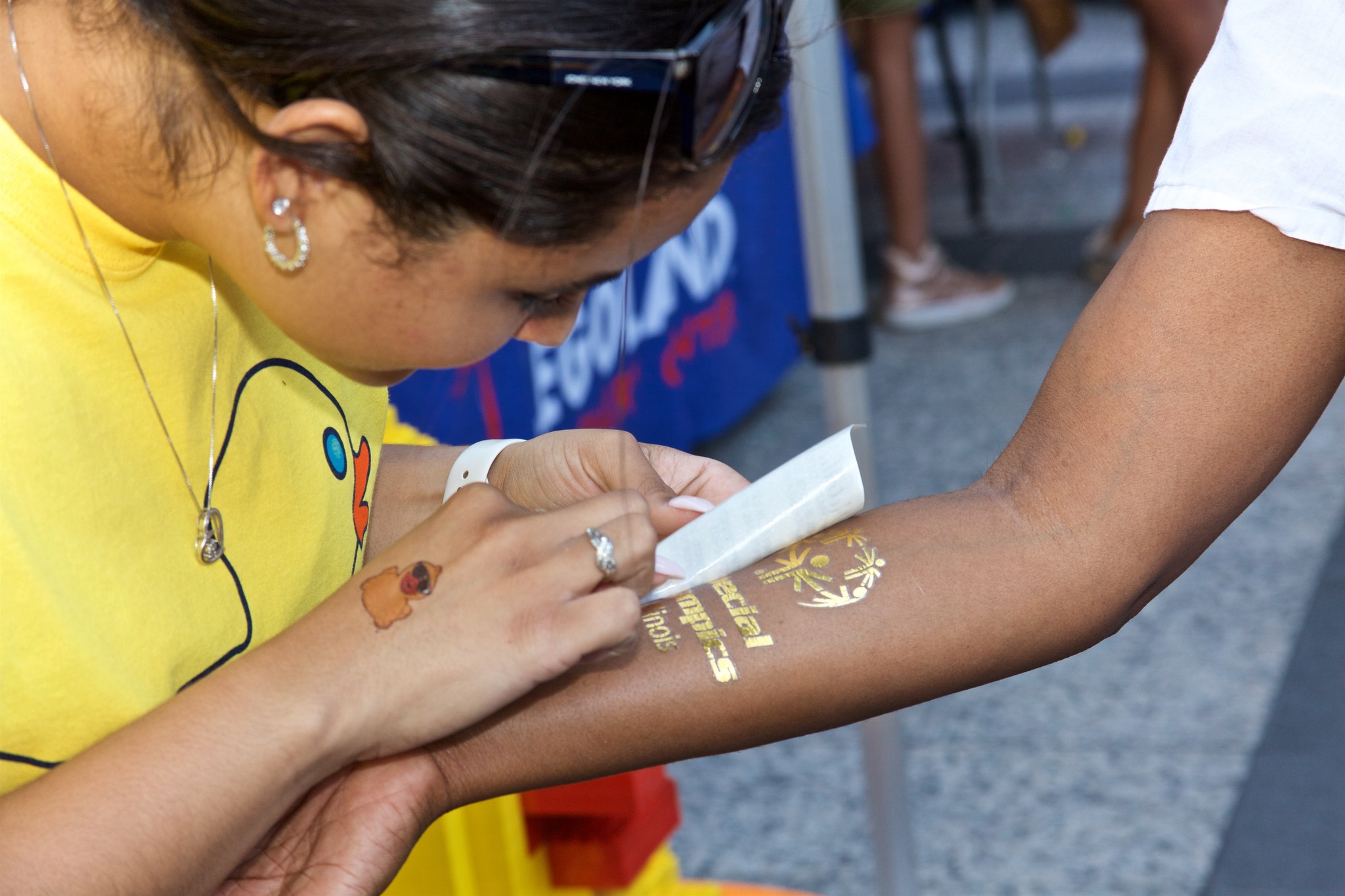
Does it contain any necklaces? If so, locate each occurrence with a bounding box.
[7,0,226,568]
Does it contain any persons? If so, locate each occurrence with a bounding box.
[1086,0,1229,287]
[843,0,1018,329]
[0,1,820,896]
[216,0,1344,896]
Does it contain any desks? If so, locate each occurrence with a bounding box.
[388,30,878,457]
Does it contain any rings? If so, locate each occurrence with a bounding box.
[587,527,620,579]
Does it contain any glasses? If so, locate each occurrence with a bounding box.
[476,0,784,174]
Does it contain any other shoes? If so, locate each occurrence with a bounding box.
[880,242,1011,331]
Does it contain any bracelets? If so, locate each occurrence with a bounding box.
[440,438,526,503]
